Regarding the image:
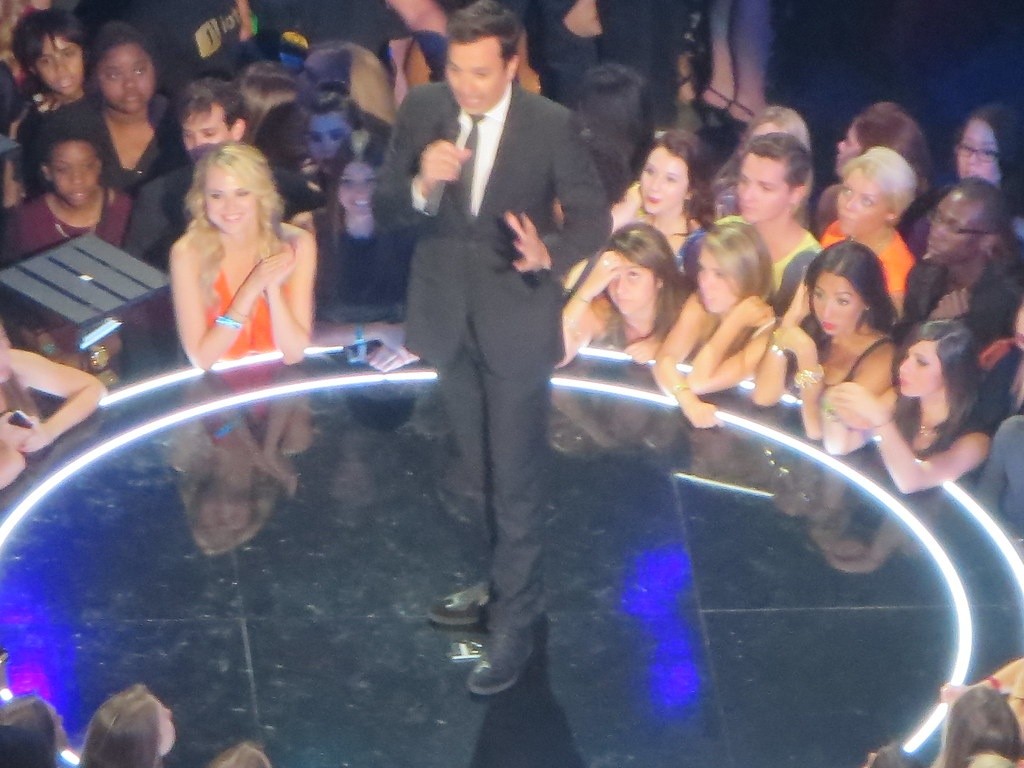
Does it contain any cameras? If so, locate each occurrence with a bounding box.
[345,340,382,365]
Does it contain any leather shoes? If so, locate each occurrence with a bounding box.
[428,580,487,626]
[467,638,534,696]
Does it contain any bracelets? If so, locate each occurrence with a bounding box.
[871,415,895,428]
[229,303,252,319]
[793,363,826,389]
[214,314,242,329]
[575,291,591,305]
[671,383,691,397]
[767,341,786,357]
[984,675,1001,693]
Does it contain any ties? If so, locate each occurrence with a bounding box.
[453,115,483,214]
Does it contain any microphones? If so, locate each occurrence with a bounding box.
[422,116,473,216]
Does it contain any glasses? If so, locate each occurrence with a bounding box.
[929,208,983,238]
[955,142,998,161]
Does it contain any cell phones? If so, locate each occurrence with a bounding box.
[8,411,33,428]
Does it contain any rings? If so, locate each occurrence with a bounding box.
[602,258,610,265]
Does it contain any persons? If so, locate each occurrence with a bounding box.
[1,681,273,768]
[522,1,1024,528]
[370,0,615,697]
[1,0,454,492]
[149,382,421,559]
[863,655,1024,768]
[544,379,930,575]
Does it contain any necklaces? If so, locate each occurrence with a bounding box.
[916,420,936,437]
